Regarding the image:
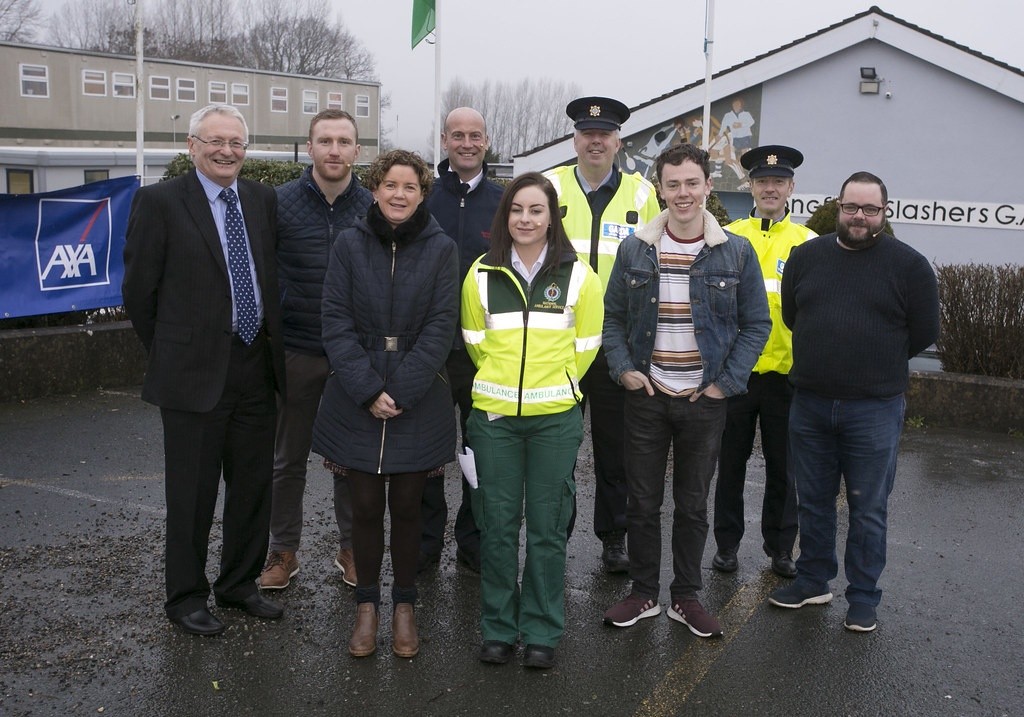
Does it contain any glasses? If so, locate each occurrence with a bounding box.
[840,203,885,216]
[191,135,247,152]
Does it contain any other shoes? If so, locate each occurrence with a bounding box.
[455,547,483,572]
[419,534,442,560]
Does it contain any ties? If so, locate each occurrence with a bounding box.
[218,188,260,348]
[460,181,470,195]
[589,190,597,203]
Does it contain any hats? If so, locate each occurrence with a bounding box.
[566,97,631,131]
[740,145,804,177]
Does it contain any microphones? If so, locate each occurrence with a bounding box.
[537,223,551,228]
[347,164,351,167]
[873,226,886,237]
[480,143,488,152]
[699,196,709,209]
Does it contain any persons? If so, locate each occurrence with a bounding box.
[461,172,604,668]
[541,96,660,574]
[416,106,505,571]
[767,171,941,632]
[314,149,460,658]
[711,144,821,576]
[603,145,773,637]
[259,109,375,589]
[122,104,282,635]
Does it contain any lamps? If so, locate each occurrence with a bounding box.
[860,67,878,78]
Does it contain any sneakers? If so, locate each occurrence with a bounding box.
[768,576,833,608]
[604,593,662,628]
[600,529,631,571]
[667,598,724,638]
[259,550,298,589]
[334,546,357,587]
[844,602,877,631]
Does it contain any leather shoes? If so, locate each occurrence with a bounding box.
[216,592,284,618]
[480,639,514,663]
[393,603,419,658]
[174,608,224,635]
[523,643,556,669]
[712,542,740,572]
[763,541,798,577]
[348,603,379,656]
